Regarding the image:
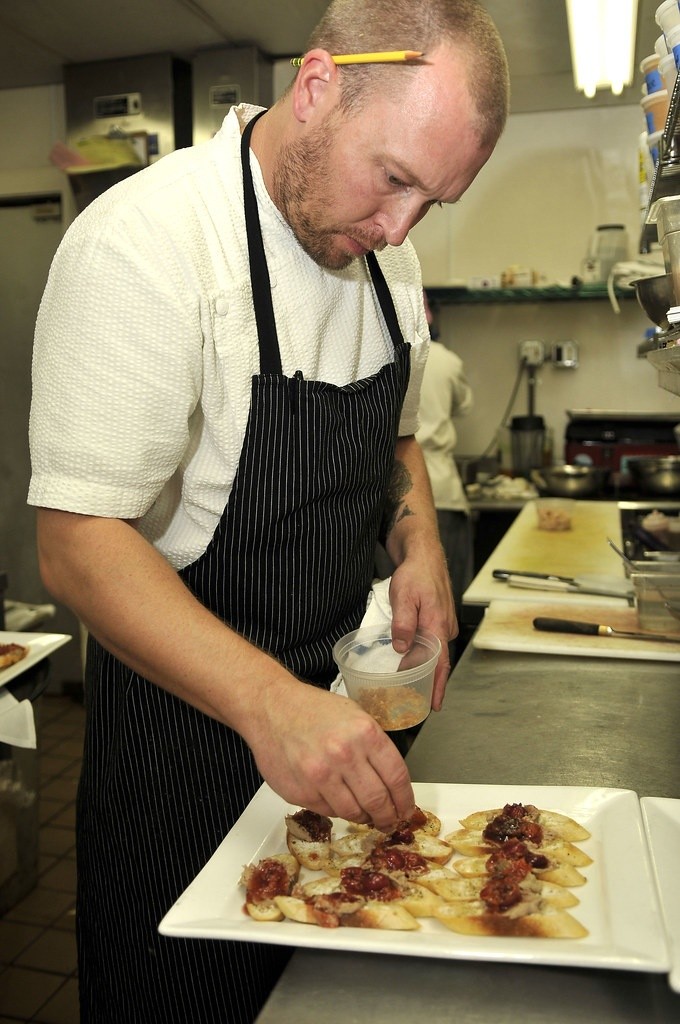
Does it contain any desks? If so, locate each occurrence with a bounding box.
[0,628,76,891]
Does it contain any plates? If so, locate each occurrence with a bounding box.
[640,797,680,994]
[0,631,73,689]
[157,780,672,975]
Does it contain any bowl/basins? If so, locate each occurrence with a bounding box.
[535,498,574,531]
[629,274,680,329]
[539,464,611,499]
[331,623,442,731]
[629,455,680,494]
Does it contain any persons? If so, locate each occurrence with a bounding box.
[27,0,511,1024]
[416,291,472,668]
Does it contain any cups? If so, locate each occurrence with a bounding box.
[637,0,680,177]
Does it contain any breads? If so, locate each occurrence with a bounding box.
[242,807,593,939]
[0,642,26,668]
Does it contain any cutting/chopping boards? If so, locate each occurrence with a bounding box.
[461,498,680,603]
[472,599,680,663]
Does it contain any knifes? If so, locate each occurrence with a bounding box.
[533,616,680,645]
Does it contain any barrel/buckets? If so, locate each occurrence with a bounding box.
[593,225,628,283]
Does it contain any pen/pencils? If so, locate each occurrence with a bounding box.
[289,49,427,68]
